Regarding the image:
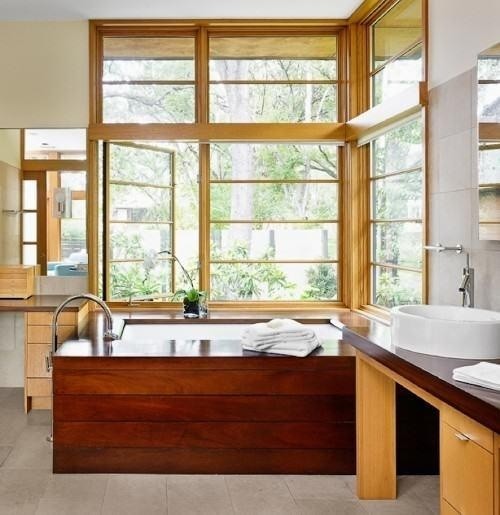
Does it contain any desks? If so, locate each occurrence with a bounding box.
[1,294,92,414]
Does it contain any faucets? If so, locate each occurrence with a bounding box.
[422,242,475,309]
[45,292,121,444]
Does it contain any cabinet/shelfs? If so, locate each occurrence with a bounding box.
[441,411,494,515]
[52,323,439,476]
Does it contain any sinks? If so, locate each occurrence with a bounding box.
[390,304,500,360]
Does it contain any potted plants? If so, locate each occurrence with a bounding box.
[170,288,208,319]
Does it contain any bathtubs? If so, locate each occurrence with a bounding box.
[117,315,346,343]
